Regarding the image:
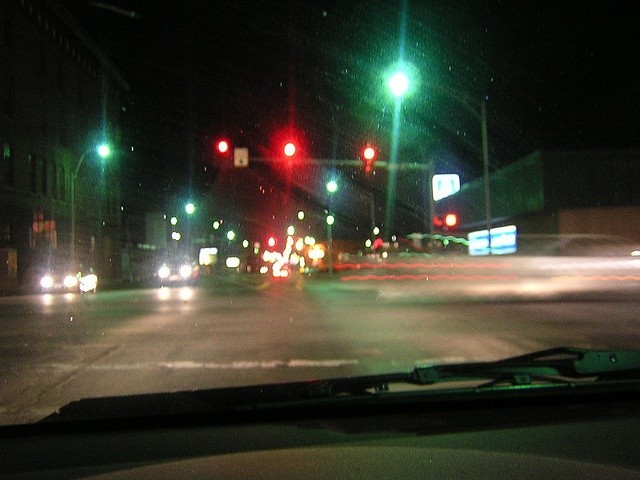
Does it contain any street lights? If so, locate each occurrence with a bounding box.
[70,142,111,274]
[387,66,492,230]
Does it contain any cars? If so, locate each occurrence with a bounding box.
[41,264,98,295]
[156,257,200,286]
